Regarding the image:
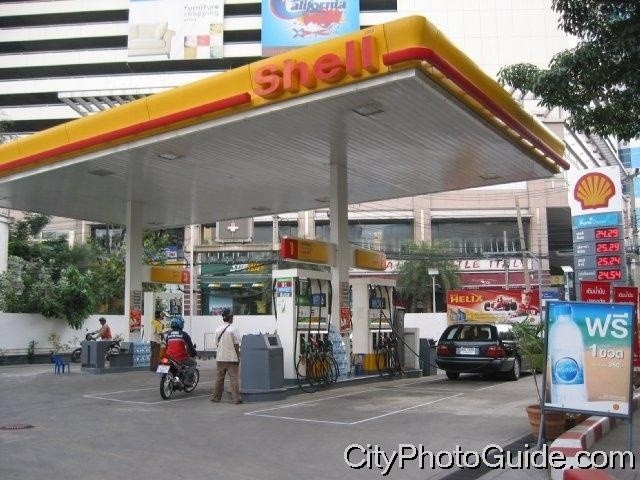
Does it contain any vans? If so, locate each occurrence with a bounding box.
[428,321,546,380]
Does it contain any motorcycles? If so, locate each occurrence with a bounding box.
[158,332,200,400]
[72,328,125,363]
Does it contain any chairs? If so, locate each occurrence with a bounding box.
[52,355,71,374]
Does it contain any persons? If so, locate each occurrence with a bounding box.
[208,309,246,404]
[148,311,171,371]
[165,314,199,389]
[511,290,540,318]
[89,317,113,341]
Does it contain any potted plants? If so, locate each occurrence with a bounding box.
[509,312,567,441]
[47,332,62,362]
[26,339,39,364]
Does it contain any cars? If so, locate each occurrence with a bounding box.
[484,295,517,312]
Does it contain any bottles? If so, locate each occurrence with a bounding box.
[547,305,589,409]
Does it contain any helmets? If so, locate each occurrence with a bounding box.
[99,317,106,321]
[171,317,183,328]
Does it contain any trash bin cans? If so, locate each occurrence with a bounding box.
[419,338,438,377]
[238,333,287,403]
